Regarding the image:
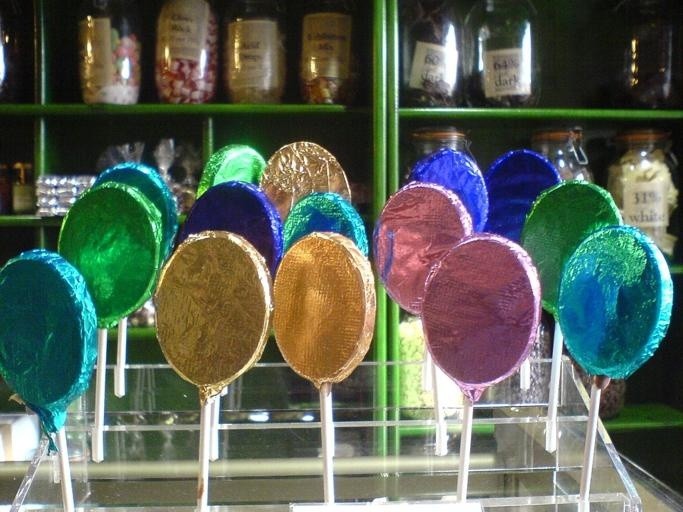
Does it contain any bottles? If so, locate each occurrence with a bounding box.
[401,1,542,106]
[1,162,35,214]
[604,0,674,110]
[77,0,362,103]
[411,124,683,263]
[394,310,627,423]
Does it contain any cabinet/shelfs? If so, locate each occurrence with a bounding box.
[386,1,681,458]
[3,1,387,460]
